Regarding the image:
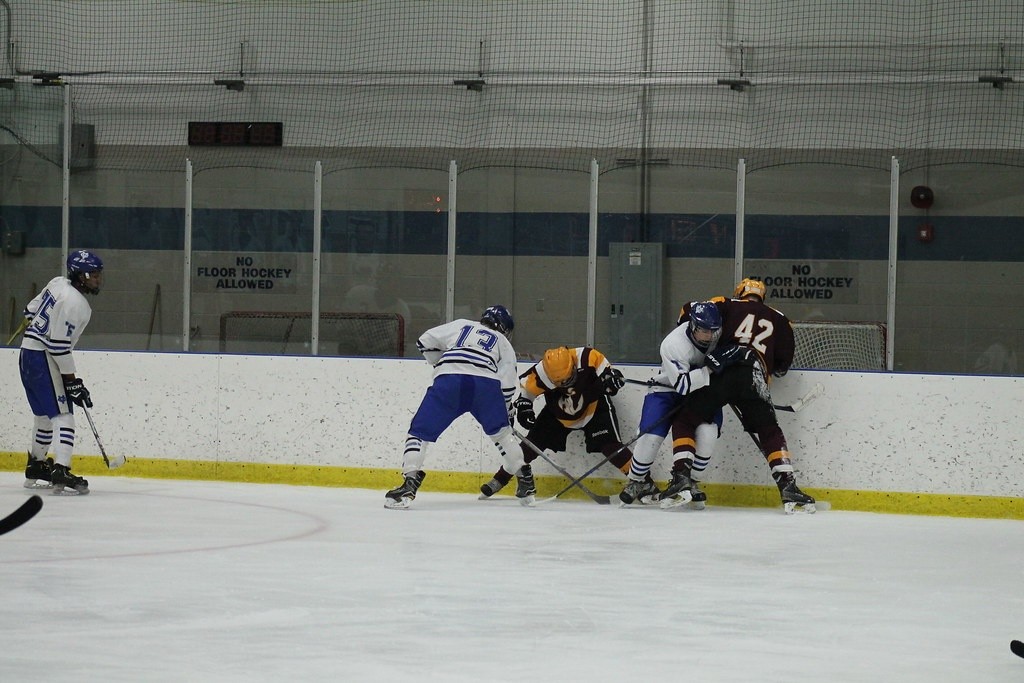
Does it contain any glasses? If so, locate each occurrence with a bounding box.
[694,325,722,342]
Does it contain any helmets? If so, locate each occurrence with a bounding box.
[732,277,767,303]
[66,250,104,295]
[542,345,579,389]
[481,305,515,343]
[687,301,723,356]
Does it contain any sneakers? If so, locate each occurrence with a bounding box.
[478,465,514,500]
[516,464,536,507]
[619,463,707,512]
[23,449,90,496]
[771,471,817,514]
[384,470,426,510]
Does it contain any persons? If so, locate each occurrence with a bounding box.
[618,278,816,514]
[18,250,105,497]
[478,345,661,501]
[384,305,537,510]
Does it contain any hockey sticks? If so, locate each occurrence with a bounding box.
[531,403,683,505]
[619,377,826,414]
[81,398,127,470]
[0,493,44,534]
[144,282,160,351]
[519,429,621,507]
[6,281,37,346]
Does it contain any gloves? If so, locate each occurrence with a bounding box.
[64,377,93,408]
[513,398,536,430]
[704,339,742,375]
[598,366,625,396]
[508,402,516,428]
